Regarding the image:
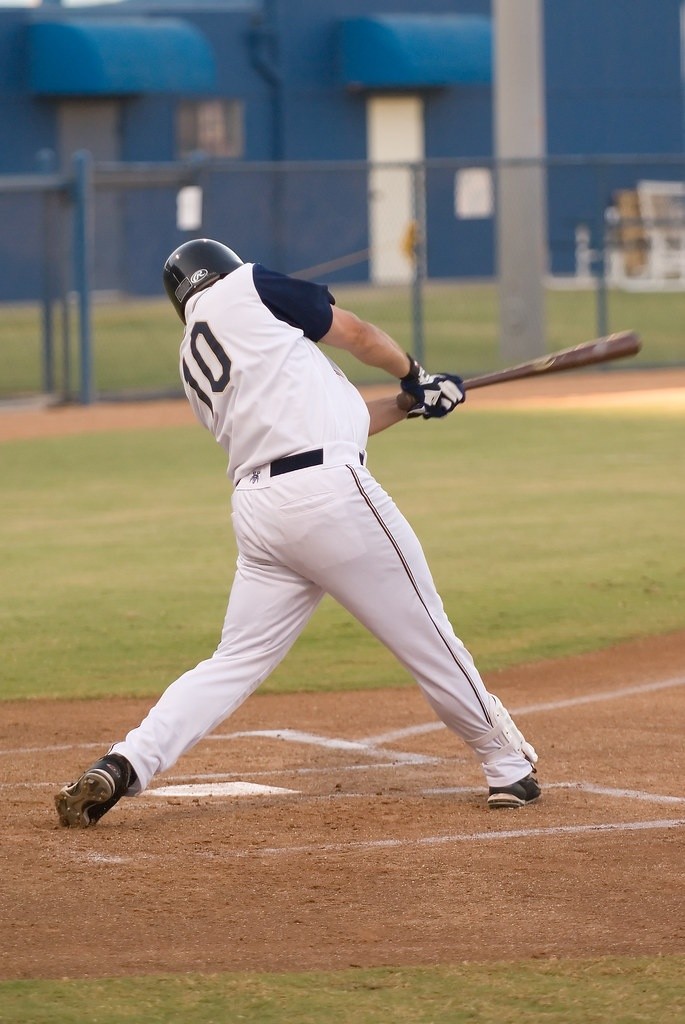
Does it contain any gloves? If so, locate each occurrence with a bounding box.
[399,352,465,419]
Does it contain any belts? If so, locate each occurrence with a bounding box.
[270,448,364,476]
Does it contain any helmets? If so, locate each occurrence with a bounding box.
[162,238,244,325]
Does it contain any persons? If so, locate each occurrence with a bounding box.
[50,239,540,832]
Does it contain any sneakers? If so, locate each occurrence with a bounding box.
[488,775,542,808]
[55,754,131,828]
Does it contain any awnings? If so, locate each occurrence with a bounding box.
[340,14,495,93]
[24,17,218,101]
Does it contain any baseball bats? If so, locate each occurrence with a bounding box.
[397,330,641,412]
